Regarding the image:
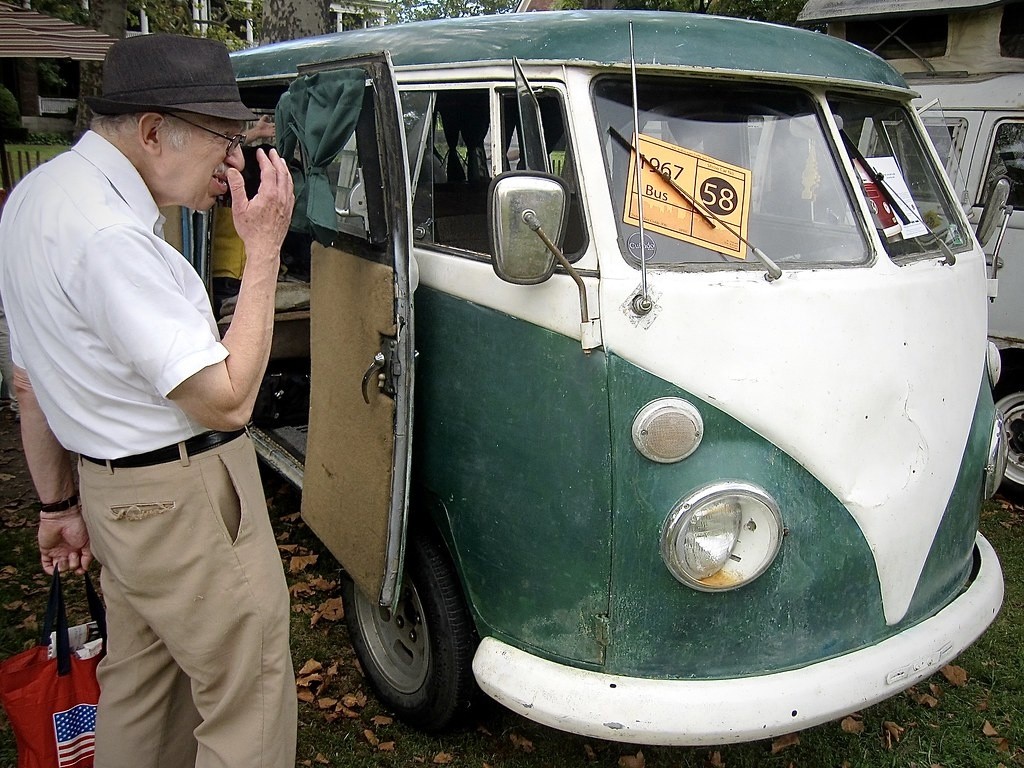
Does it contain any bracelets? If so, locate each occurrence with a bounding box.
[40,493,79,513]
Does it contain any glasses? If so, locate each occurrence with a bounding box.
[163,111,246,157]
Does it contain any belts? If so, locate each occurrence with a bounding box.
[81,424,251,468]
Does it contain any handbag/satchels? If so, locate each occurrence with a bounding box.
[0,561,108,768]
[252,357,311,430]
[211,207,246,280]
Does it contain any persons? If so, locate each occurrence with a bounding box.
[0,33,298,768]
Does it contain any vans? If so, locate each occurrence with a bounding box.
[747,68,1023,506]
[160,8,1008,746]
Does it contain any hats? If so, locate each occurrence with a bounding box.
[84,34,260,121]
